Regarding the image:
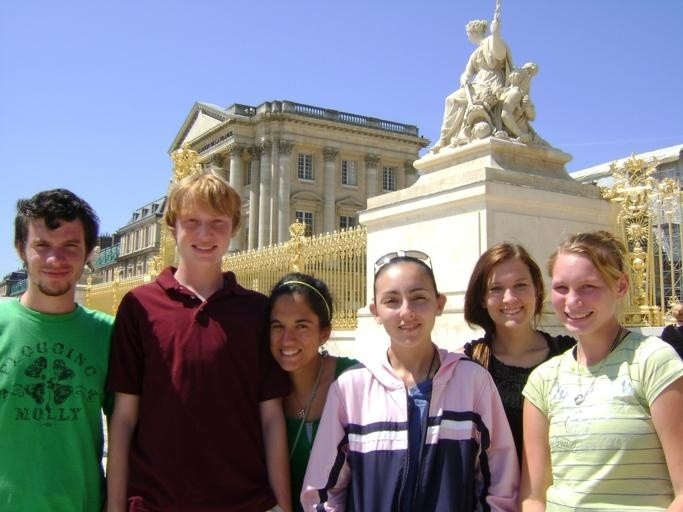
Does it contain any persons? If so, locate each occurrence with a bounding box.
[456,243,577,467]
[430,0,552,153]
[0,184,117,511]
[518,231,683,512]
[300,249,518,511]
[104,172,292,511]
[269,272,366,511]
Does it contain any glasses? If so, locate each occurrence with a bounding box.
[374,250,432,280]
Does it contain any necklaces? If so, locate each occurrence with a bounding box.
[573,326,621,406]
[295,397,310,422]
